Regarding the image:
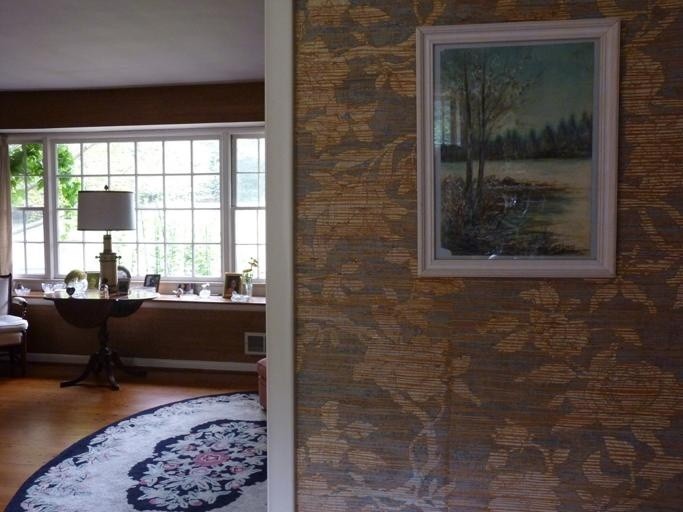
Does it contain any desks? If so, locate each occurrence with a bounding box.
[48,288,159,389]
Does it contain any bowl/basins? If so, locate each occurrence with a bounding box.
[40,280,67,291]
[13,287,33,296]
[127,285,158,300]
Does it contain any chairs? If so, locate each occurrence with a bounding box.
[0,273,27,379]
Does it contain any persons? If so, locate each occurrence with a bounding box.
[225,278,239,295]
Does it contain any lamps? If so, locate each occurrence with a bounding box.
[77,183,134,292]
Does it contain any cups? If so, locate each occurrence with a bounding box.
[117,277,131,296]
[53,288,67,298]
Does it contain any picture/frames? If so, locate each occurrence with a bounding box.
[223,272,243,298]
[416,23,617,280]
[143,274,160,291]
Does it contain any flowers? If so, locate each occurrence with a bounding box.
[242,257,259,283]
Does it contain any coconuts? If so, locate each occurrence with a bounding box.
[64,270,88,285]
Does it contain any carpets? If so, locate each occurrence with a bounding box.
[2,390,265,512]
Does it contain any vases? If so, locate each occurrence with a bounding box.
[245,277,253,301]
